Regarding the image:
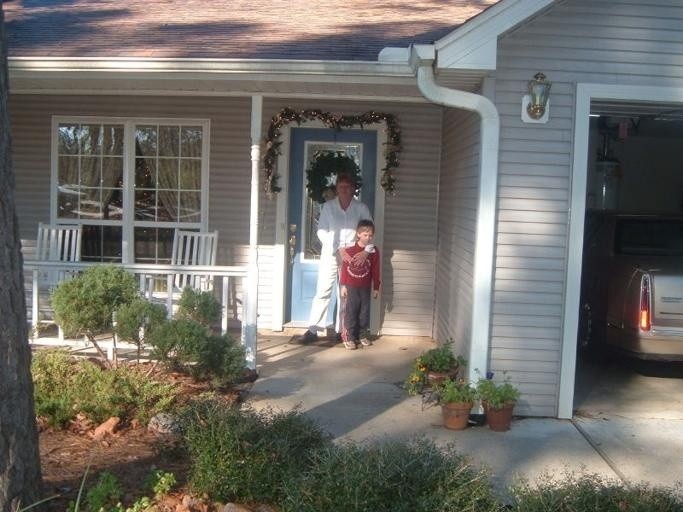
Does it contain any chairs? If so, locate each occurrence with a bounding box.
[22,221,82,340]
[138,228,219,344]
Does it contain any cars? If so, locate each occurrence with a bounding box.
[576,209,683,363]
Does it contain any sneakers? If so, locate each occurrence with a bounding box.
[299,330,317,342]
[336,332,372,349]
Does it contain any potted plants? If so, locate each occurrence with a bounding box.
[475,368,520,431]
[431,378,476,430]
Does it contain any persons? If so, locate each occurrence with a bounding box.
[339,220,380,350]
[295,171,373,344]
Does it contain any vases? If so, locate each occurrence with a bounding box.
[426,369,459,387]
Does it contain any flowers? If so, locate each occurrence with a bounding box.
[408,336,469,396]
[261,109,402,200]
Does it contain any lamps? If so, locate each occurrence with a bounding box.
[520,72,552,123]
